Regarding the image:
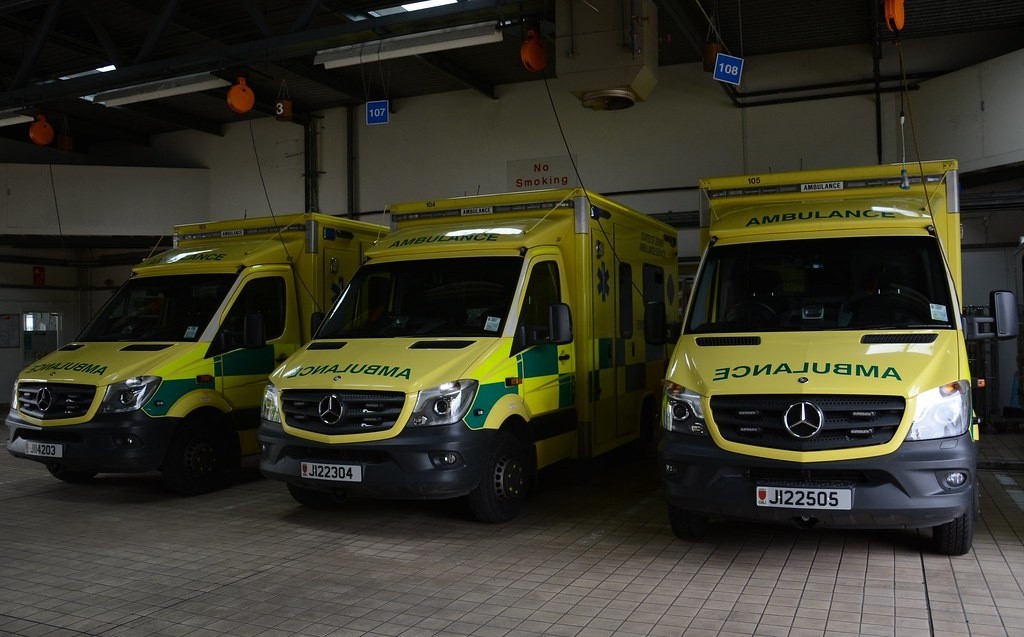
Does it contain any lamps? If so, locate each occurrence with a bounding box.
[93,68,233,107]
[0,106,35,128]
[313,20,504,69]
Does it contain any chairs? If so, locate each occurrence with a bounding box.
[221,282,254,325]
[735,251,785,328]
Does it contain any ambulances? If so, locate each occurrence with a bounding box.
[257,184,682,526]
[643,158,1019,556]
[4,210,396,499]
